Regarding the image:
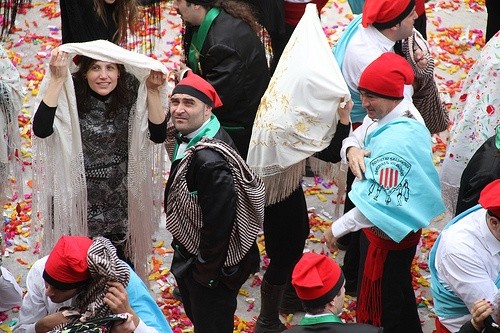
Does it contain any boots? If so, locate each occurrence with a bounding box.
[280,280,303,314]
[254,276,288,333]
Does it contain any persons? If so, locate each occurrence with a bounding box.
[428,179,500,333]
[0,264,24,311]
[332,0,430,333]
[162,71,266,333]
[59,0,139,48]
[12,235,174,333]
[278,253,384,333]
[323,52,446,333]
[455,124,500,218]
[237,52,357,333]
[30,39,169,290]
[173,0,281,162]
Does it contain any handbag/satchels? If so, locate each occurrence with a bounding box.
[46,305,128,333]
[166,136,264,267]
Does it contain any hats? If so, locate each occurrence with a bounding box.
[478,179,500,221]
[357,52,415,100]
[362,0,415,29]
[291,252,344,304]
[43,236,92,290]
[172,70,223,110]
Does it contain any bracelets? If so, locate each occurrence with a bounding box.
[148,87,160,94]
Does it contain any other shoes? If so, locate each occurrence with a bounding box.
[346,279,359,294]
[172,286,182,301]
